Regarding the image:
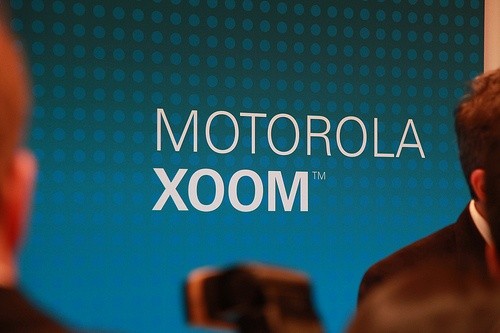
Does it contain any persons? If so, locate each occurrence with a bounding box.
[0,8,87,333]
[338,66,500,333]
